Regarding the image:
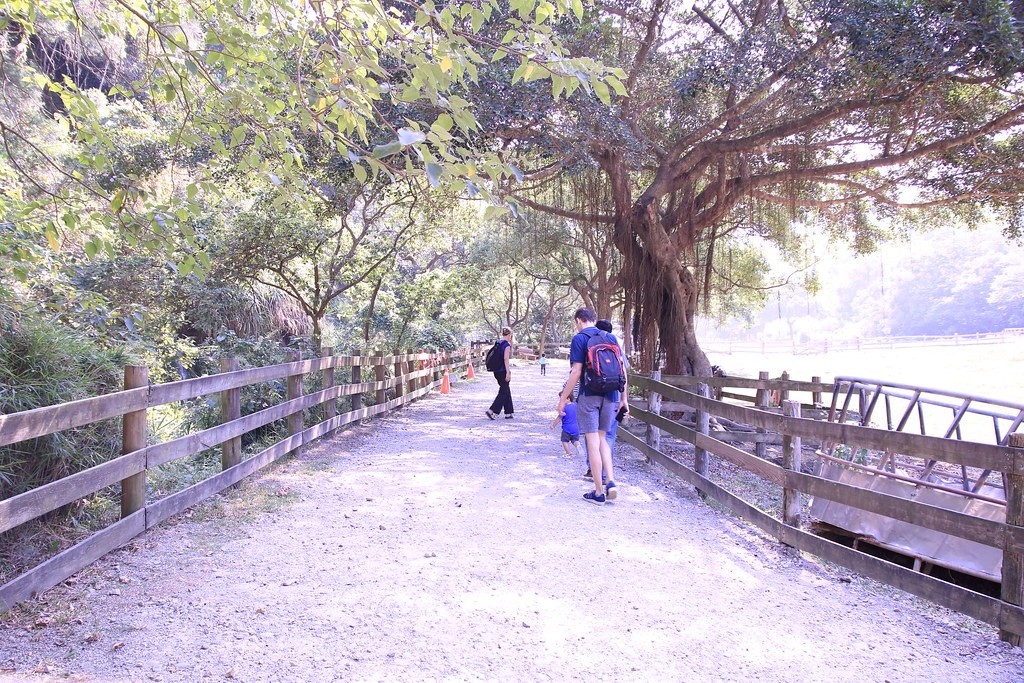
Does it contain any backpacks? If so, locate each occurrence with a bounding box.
[578,330,626,395]
[486,340,507,371]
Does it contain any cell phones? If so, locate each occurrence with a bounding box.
[615,407,628,423]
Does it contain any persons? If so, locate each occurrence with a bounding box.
[485,327,515,420]
[549,307,629,507]
[539,353,547,376]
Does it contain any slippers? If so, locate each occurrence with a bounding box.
[505,414,513,419]
[486,411,496,420]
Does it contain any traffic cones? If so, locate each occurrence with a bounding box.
[466,362,475,379]
[441,367,453,394]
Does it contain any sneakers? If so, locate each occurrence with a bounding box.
[582,488,605,505]
[605,481,617,500]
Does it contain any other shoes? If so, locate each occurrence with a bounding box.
[576,445,584,455]
[562,453,573,459]
[601,474,606,485]
[583,470,593,479]
